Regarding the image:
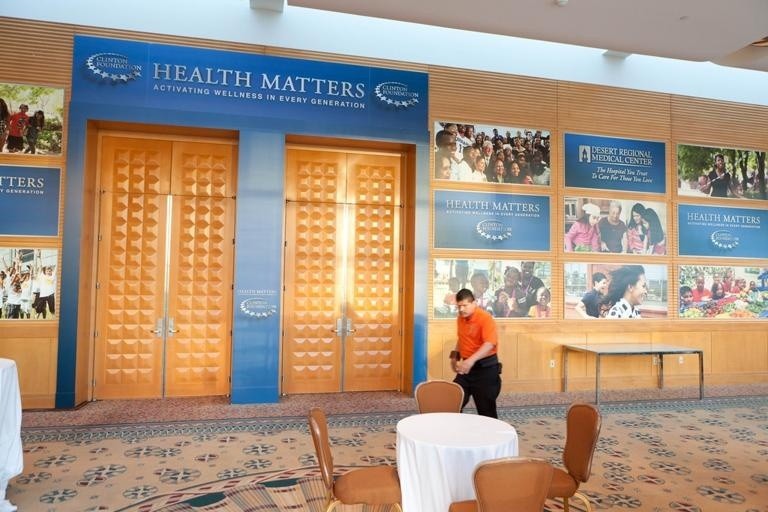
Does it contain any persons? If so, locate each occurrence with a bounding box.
[640,208,666,255]
[595,265,647,319]
[699,153,745,199]
[434,260,551,318]
[627,203,646,254]
[23,110,44,155]
[565,203,600,252]
[7,103,30,153]
[574,271,608,319]
[449,287,503,419]
[435,123,551,186]
[0,247,56,320]
[678,267,756,312]
[599,201,629,254]
[1,98,10,152]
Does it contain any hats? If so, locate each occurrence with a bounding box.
[582,203,600,216]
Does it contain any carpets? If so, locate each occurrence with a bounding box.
[7,395,768,512]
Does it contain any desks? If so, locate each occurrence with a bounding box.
[396,412,518,512]
[0,357,24,512]
[562,345,704,404]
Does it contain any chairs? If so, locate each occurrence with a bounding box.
[449,457,552,512]
[536,402,601,512]
[414,380,465,413]
[308,409,401,512]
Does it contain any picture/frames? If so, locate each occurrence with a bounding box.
[678,267,764,319]
[0,245,58,322]
[0,163,62,239]
[0,81,65,158]
[432,189,551,253]
[677,204,768,259]
[432,257,552,319]
[563,196,668,255]
[563,131,667,194]
[563,262,668,320]
[676,142,768,201]
[434,119,551,187]
